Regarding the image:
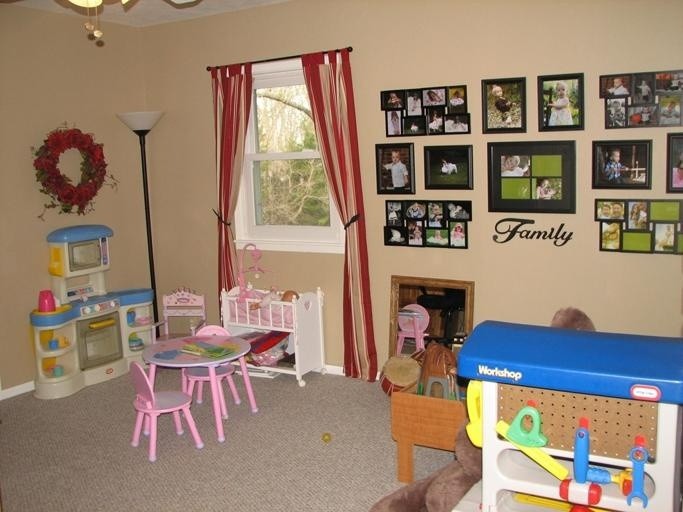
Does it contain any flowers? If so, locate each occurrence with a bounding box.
[30,123,120,221]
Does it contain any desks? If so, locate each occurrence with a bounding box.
[142,336,260,443]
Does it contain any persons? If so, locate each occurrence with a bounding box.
[636,79,652,103]
[656,225,673,249]
[599,204,611,220]
[434,230,443,241]
[602,222,619,233]
[384,92,402,108]
[546,82,574,125]
[449,91,464,106]
[426,90,442,103]
[452,224,465,244]
[412,226,421,244]
[605,149,629,185]
[545,188,558,198]
[491,84,517,124]
[630,203,647,226]
[620,102,626,126]
[602,233,619,243]
[608,78,629,94]
[390,229,401,242]
[250,289,300,311]
[429,204,443,226]
[436,114,443,126]
[448,203,469,218]
[660,101,679,123]
[658,80,683,93]
[611,102,623,126]
[390,111,399,133]
[639,106,652,124]
[502,157,530,176]
[537,179,549,199]
[427,111,438,133]
[407,93,420,114]
[452,115,465,131]
[672,152,683,188]
[611,203,624,219]
[384,149,408,192]
[407,120,418,132]
[388,206,397,224]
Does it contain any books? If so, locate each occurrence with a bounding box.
[180,341,235,360]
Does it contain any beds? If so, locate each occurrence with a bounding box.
[223,285,326,389]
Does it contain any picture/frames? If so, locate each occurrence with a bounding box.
[481,77,527,134]
[384,199,471,249]
[423,144,475,191]
[593,198,682,256]
[486,139,576,214]
[380,85,471,138]
[665,132,682,193]
[598,70,682,129]
[375,143,415,194]
[591,139,652,190]
[389,273,474,358]
[537,73,584,132]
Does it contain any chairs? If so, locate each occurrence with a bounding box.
[184,326,242,420]
[130,362,205,461]
